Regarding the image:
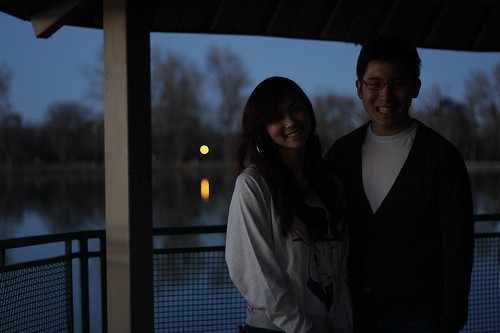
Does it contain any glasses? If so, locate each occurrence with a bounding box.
[358,78,416,90]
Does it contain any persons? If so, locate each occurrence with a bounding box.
[321,35,475,333]
[224,76,354,333]
